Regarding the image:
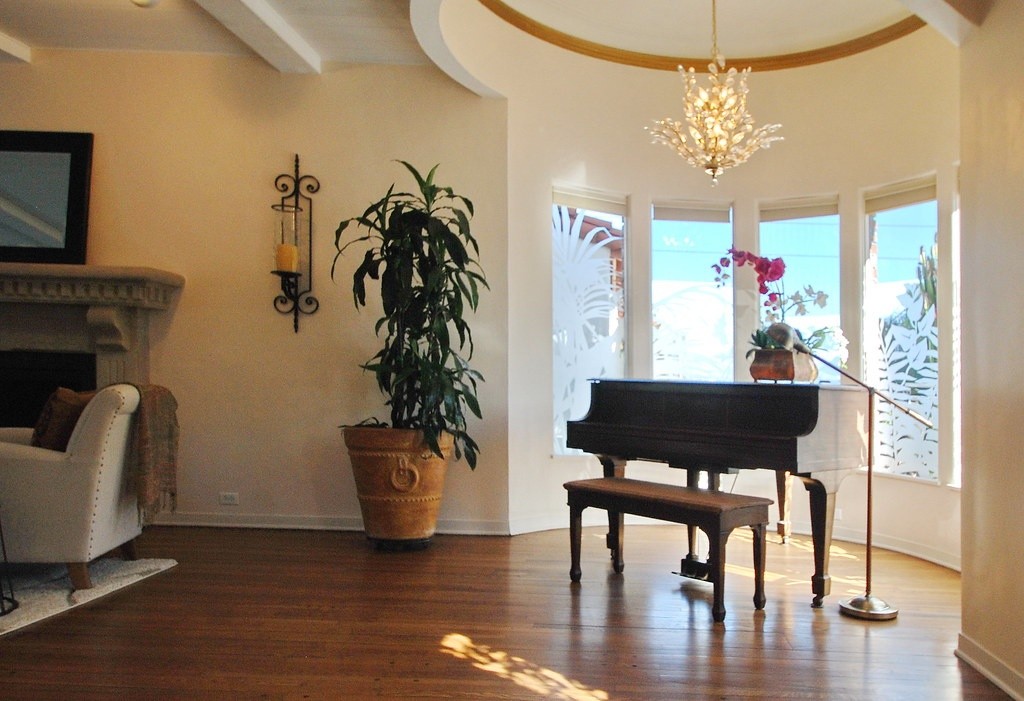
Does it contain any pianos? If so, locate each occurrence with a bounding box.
[567,376,869,609]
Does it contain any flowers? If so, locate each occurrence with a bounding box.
[713,244,850,369]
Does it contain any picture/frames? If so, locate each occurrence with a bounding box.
[1,128,93,266]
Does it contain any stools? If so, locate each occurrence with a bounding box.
[563,475,777,623]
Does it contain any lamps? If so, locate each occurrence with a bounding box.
[640,1,783,189]
[269,148,325,335]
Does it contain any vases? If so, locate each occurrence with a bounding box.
[746,349,818,386]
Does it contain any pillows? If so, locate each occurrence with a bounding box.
[31,386,105,455]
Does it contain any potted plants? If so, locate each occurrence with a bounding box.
[338,158,492,541]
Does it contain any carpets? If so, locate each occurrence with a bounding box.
[1,559,180,638]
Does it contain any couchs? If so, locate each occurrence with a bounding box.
[0,382,176,588]
